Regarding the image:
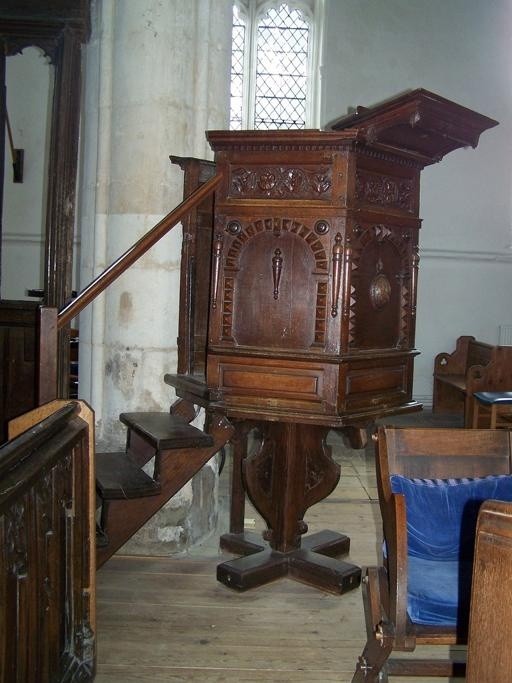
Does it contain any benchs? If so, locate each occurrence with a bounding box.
[430,333,512,428]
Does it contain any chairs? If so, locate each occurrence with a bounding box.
[348,423,512,682]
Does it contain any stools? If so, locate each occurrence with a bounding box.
[471,389,512,430]
